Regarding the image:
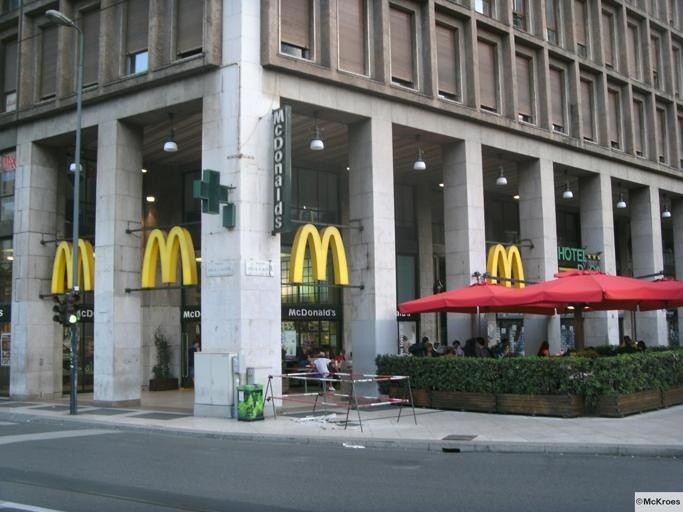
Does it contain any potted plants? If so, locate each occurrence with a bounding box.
[374,348,683,419]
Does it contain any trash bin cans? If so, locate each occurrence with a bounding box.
[237,384,264,422]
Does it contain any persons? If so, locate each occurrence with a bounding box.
[623,336,645,352]
[184,339,202,388]
[537,341,550,357]
[283,344,353,391]
[407,336,512,357]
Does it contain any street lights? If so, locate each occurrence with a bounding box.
[43,8,85,416]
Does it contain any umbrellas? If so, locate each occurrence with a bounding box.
[399,270,683,342]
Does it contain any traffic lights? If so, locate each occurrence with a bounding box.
[53,293,80,327]
